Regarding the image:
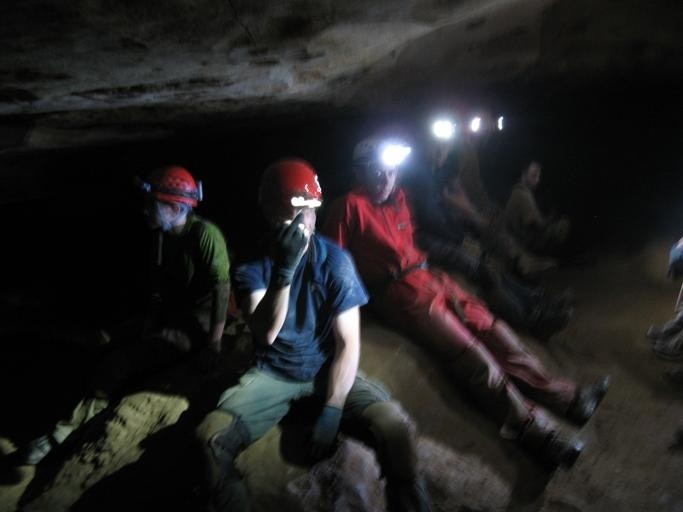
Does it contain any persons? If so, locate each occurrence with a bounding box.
[643,234,683,365]
[394,110,607,336]
[1,165,234,502]
[311,130,612,460]
[659,365,683,453]
[188,155,444,510]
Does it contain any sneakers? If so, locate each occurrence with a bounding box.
[527,250,575,339]
[646,313,683,385]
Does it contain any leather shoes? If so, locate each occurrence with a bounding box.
[577,371,617,432]
[548,428,592,475]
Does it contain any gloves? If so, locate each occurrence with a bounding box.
[301,405,341,459]
[273,213,309,277]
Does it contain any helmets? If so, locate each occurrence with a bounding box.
[352,108,504,166]
[260,158,322,201]
[153,166,202,207]
